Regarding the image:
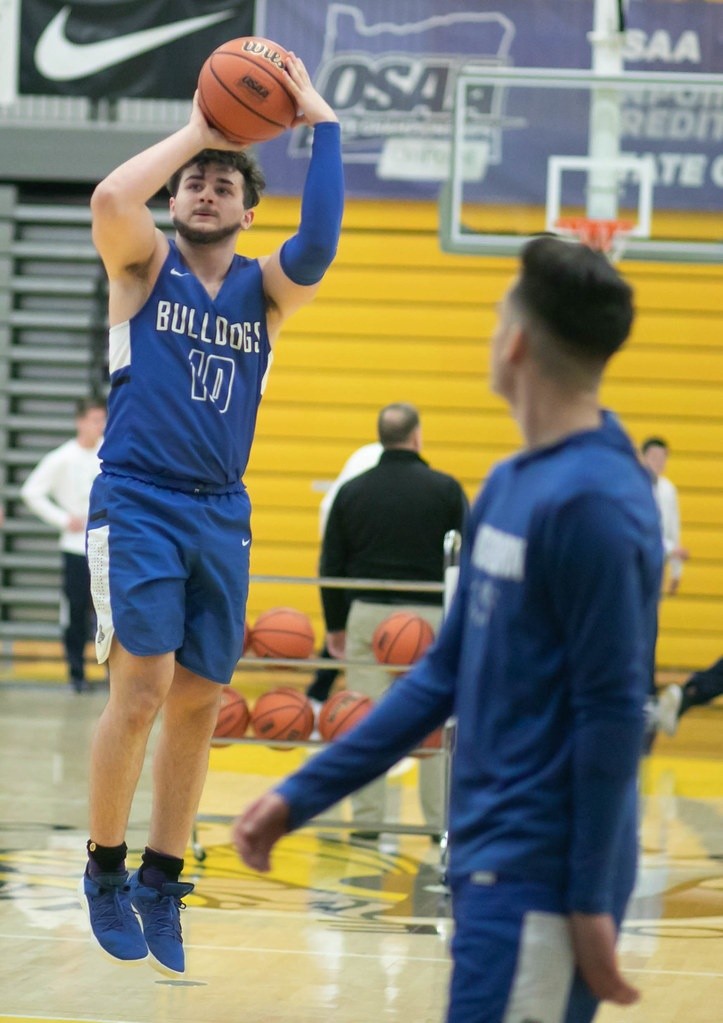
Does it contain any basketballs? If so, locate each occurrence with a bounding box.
[209,686,250,748]
[251,607,313,671]
[319,690,378,746]
[251,689,317,751]
[374,614,437,674]
[197,35,298,146]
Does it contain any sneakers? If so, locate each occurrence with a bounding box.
[127,867,194,972]
[80,859,149,960]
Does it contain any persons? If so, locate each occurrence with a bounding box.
[21,397,109,693]
[82,50,344,978]
[310,403,723,842]
[234,238,667,1023]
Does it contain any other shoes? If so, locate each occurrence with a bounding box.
[656,683,683,737]
[70,674,86,692]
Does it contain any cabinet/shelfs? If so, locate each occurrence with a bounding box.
[192,527,460,890]
